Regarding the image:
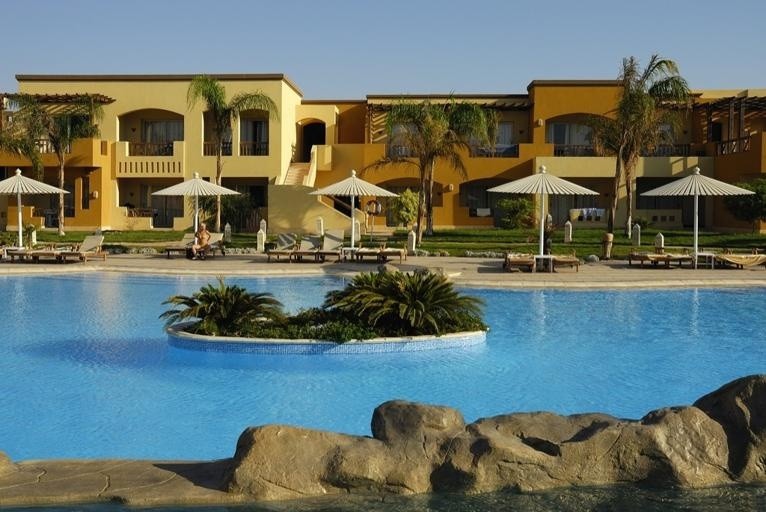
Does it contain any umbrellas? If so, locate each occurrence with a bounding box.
[150,171,242,245]
[0,169,71,247]
[640,166,756,263]
[307,169,402,248]
[486,165,600,255]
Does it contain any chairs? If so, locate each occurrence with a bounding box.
[264,229,409,264]
[506,250,580,273]
[630,246,766,270]
[0,235,107,264]
[164,232,226,258]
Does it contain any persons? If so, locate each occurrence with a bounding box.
[191,223,211,259]
